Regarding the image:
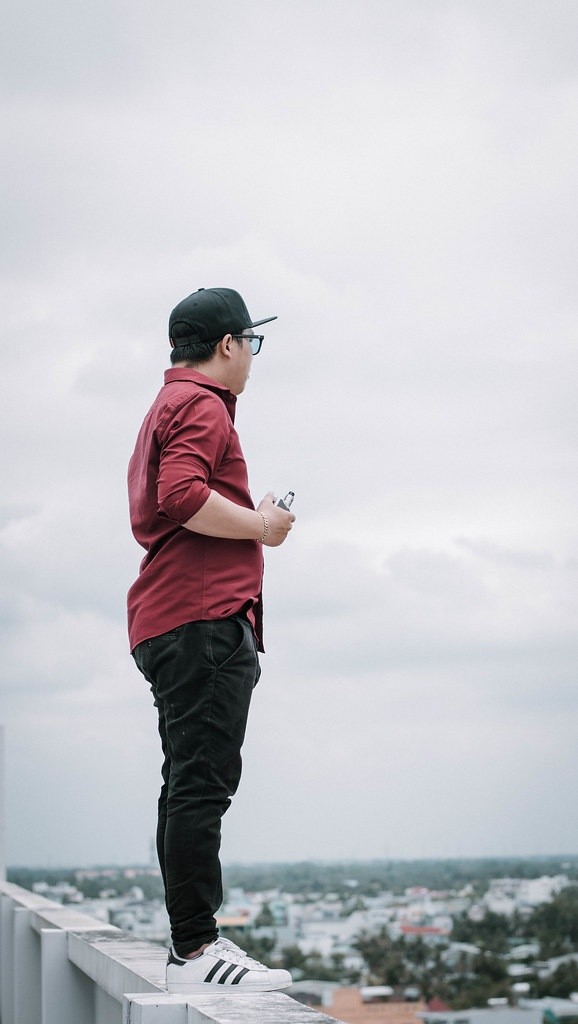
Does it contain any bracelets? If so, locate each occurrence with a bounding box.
[255,510,270,543]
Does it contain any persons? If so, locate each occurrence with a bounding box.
[126,287,295,996]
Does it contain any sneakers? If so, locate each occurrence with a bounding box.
[166,937,292,991]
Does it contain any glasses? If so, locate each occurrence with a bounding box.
[211,335,264,355]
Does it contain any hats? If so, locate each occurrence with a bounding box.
[169,288,278,348]
